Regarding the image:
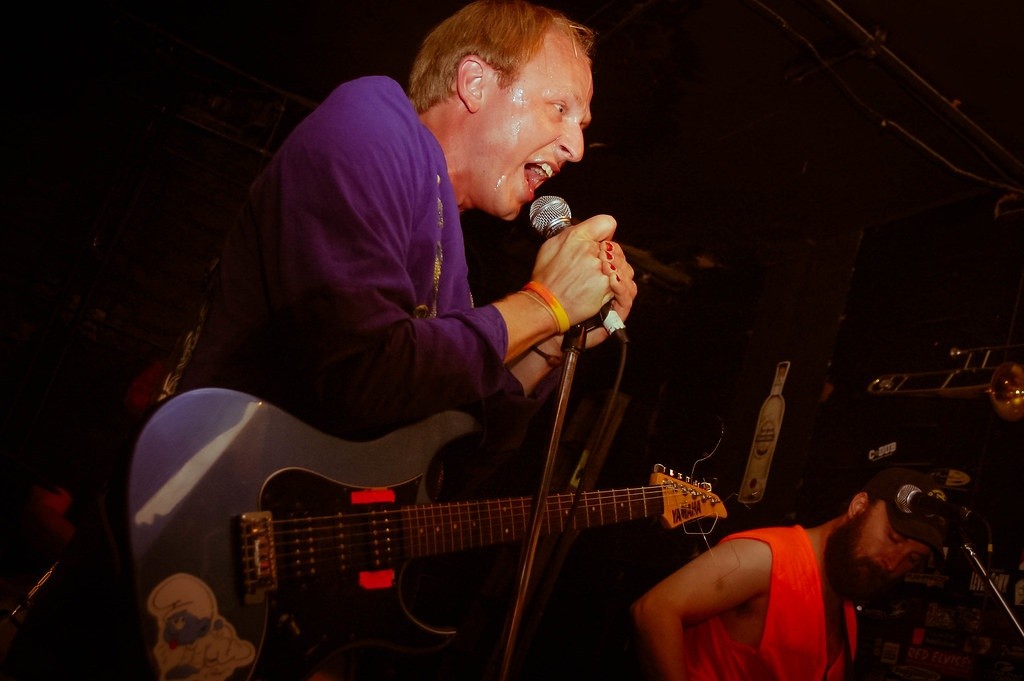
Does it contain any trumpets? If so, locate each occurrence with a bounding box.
[863,339,1024,425]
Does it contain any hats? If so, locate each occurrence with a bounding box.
[874,468,946,552]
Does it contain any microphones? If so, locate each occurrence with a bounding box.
[530,195,631,346]
[896,485,972,521]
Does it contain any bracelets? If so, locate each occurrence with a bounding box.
[522,281,572,334]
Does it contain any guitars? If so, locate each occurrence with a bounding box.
[120,384,729,681]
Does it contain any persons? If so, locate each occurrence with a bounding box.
[631,467,948,681]
[136,0,637,680]
